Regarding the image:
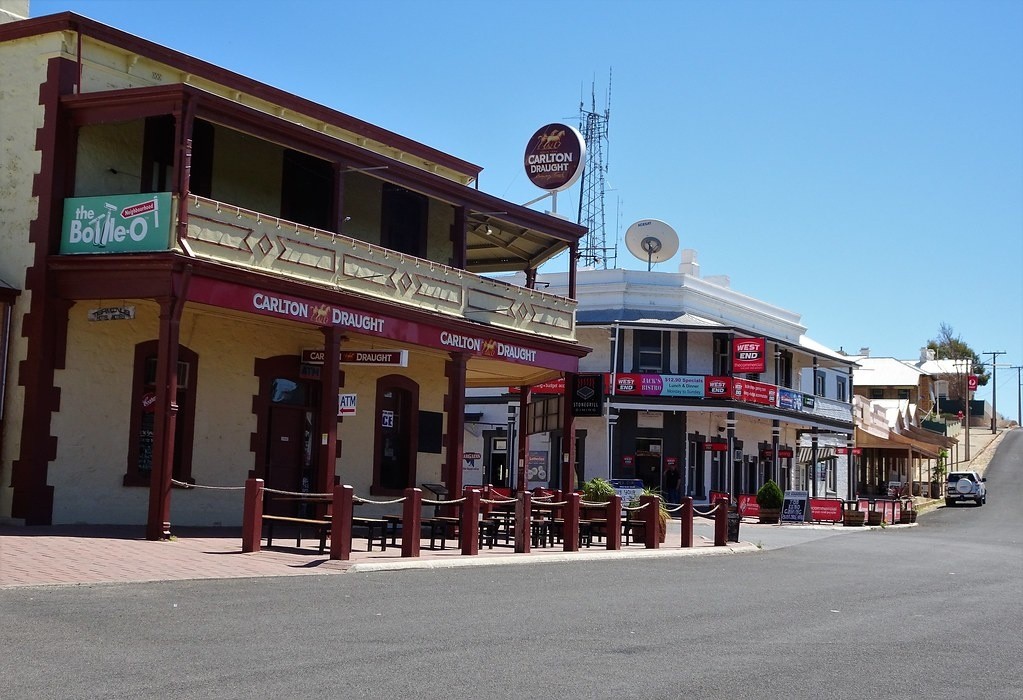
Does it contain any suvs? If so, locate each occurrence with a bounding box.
[942,471,986,506]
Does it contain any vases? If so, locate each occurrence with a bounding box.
[900,511,917,524]
[844,510,865,527]
[868,511,882,526]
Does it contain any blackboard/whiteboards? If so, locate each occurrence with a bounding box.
[608,478,644,516]
[781,490,809,522]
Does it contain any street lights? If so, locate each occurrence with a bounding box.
[964,362,1012,461]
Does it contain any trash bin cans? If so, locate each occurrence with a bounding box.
[930,479,940,499]
[727,509,741,542]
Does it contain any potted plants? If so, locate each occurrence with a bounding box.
[931,450,948,499]
[628,485,670,544]
[580,478,620,535]
[756,479,783,524]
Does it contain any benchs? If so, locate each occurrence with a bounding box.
[261,509,647,555]
